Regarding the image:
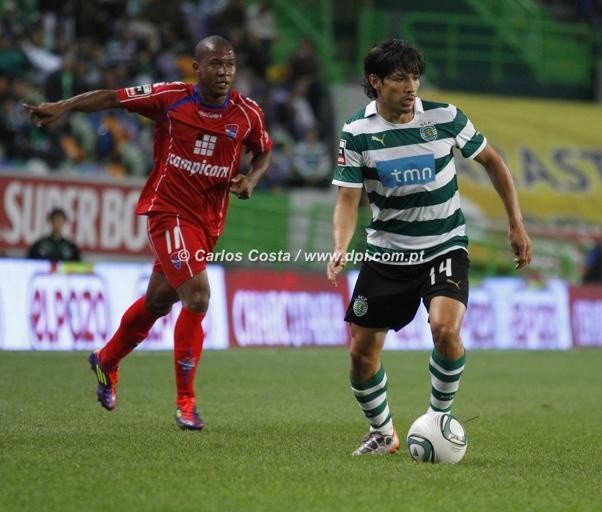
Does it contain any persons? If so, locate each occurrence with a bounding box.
[24,36,273,432]
[0,0,370,206]
[27,209,81,260]
[327,38,533,457]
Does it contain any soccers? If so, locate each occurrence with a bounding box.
[408,412,467,464]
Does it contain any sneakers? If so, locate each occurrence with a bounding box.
[353,426,400,455]
[175,397,203,429]
[89,350,119,411]
